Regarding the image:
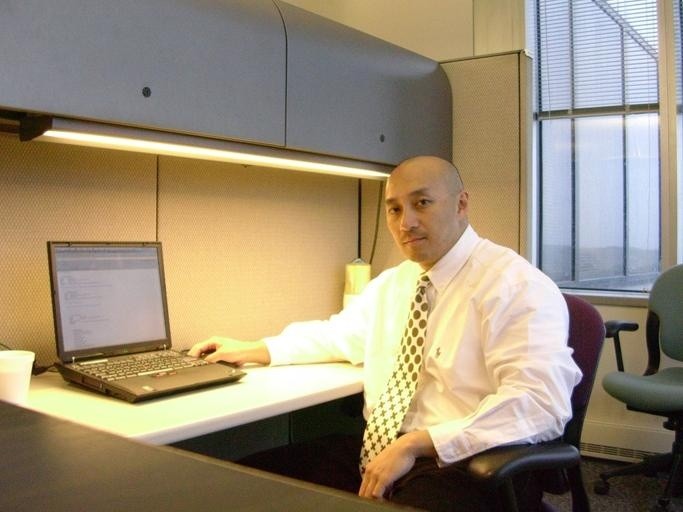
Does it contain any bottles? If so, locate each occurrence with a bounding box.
[343,258,373,309]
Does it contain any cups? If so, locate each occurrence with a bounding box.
[1,348,36,404]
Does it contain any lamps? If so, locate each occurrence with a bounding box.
[16,114,392,190]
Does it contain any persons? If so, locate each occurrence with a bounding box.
[183,153,583,510]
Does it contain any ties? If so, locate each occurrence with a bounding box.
[358,272,433,480]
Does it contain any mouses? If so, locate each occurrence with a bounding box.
[183,348,242,368]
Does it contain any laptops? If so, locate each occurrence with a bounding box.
[46,241,247,403]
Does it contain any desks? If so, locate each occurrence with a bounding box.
[16,346,362,445]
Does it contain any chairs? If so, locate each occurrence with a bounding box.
[414,290,605,511]
[592,264,682,507]
